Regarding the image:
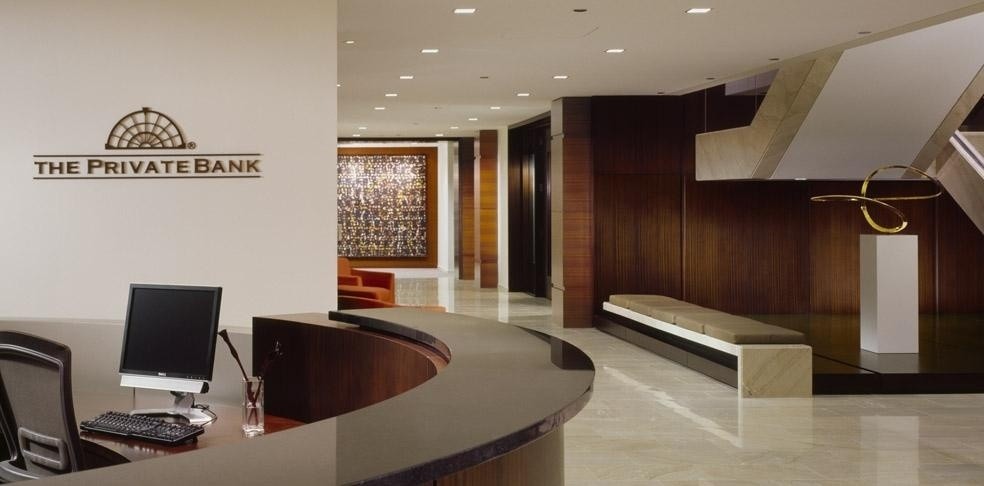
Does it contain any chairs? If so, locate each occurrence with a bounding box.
[1,328,92,486]
[337,256,395,307]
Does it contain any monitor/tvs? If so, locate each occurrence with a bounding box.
[117,284,223,421]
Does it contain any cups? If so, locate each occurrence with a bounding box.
[240,378,265,435]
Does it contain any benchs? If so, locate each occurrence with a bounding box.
[600,293,815,399]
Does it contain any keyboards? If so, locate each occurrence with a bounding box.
[79,410,205,447]
